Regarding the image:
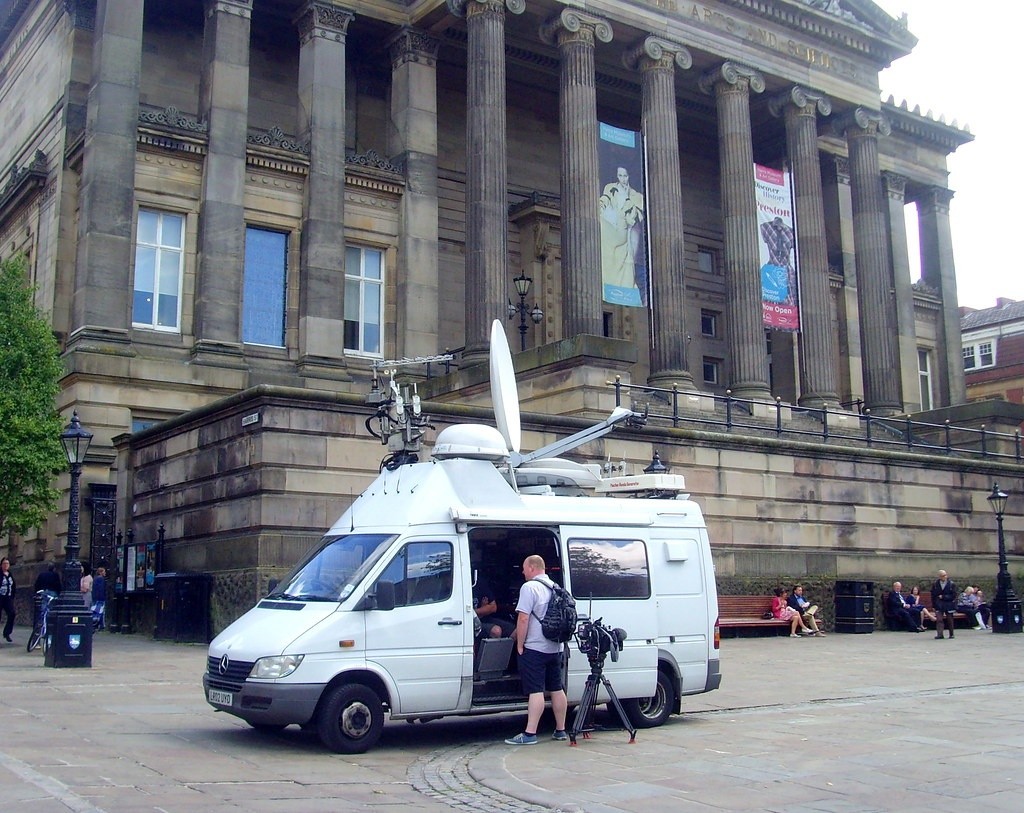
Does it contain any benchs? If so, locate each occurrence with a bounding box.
[717,595,822,637]
[881,592,968,631]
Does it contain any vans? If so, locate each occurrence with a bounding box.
[203,420,723,745]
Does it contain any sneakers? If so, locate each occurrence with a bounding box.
[504,732,537,745]
[552,730,568,741]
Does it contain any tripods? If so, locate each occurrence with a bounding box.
[568,653,637,748]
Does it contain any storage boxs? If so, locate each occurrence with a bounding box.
[473,637,515,681]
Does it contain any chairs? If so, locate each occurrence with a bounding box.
[410,565,447,606]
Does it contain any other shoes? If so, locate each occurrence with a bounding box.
[802,628,813,633]
[3,633,12,642]
[934,635,944,639]
[99,628,106,632]
[814,630,826,637]
[790,633,802,638]
[949,635,955,639]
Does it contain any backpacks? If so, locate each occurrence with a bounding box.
[525,573,577,644]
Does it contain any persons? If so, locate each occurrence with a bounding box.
[772,587,813,637]
[931,570,959,639]
[505,554,567,744]
[887,581,925,632]
[957,585,990,630]
[905,586,937,630]
[31,562,61,649]
[92,567,106,631]
[599,160,643,306]
[787,586,827,637]
[0,558,16,642]
[80,561,93,609]
[440,547,511,678]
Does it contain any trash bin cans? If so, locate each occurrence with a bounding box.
[153,570,213,644]
[834,580,876,634]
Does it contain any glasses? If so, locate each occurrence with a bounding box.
[940,573,947,577]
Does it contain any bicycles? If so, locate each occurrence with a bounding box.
[27,590,55,657]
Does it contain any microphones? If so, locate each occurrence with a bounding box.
[611,627,627,641]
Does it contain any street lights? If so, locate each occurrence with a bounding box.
[36,410,97,669]
[984,481,1023,635]
[507,269,544,352]
